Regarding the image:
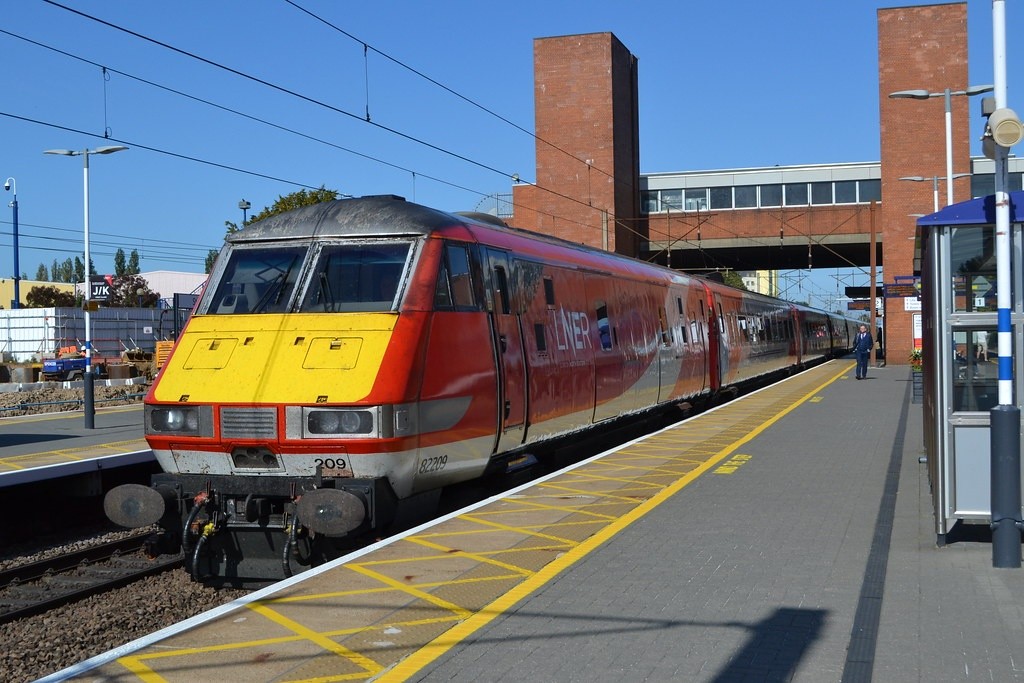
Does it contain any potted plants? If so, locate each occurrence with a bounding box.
[908,351,923,364]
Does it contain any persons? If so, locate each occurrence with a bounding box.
[876,327,882,354]
[853,324,873,380]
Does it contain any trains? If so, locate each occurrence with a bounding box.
[143,193,883,582]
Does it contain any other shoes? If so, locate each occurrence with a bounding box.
[862,377,866,380]
[856,377,861,380]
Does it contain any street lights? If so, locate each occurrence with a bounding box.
[898,173,973,213]
[42,145,131,430]
[239,201,251,222]
[889,83,995,205]
